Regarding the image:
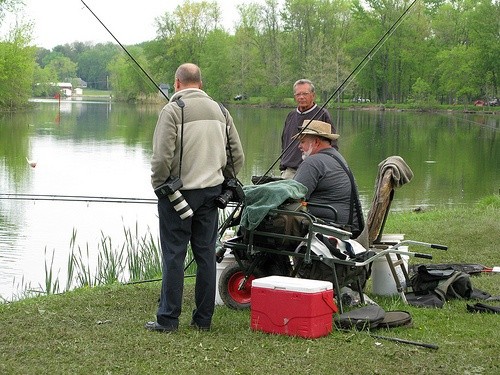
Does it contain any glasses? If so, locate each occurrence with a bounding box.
[295,126,321,134]
[295,91,311,97]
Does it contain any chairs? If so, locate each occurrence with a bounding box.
[351,155,415,246]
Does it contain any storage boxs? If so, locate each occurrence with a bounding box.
[251,275,338,340]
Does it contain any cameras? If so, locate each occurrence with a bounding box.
[216,179,246,209]
[154,175,195,222]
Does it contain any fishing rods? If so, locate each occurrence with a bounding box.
[0,192,242,208]
[80,0,170,103]
[218,1,418,235]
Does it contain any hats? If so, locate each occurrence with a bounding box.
[291,120,340,142]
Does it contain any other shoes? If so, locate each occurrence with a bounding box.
[145,320,178,332]
[186,321,211,331]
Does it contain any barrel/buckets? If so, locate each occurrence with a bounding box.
[372,246,409,296]
[214,246,237,306]
[374,233,405,245]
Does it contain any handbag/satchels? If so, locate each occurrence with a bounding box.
[409,265,473,301]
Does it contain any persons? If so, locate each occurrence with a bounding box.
[279,79,340,179]
[147,63,245,332]
[291,119,365,240]
